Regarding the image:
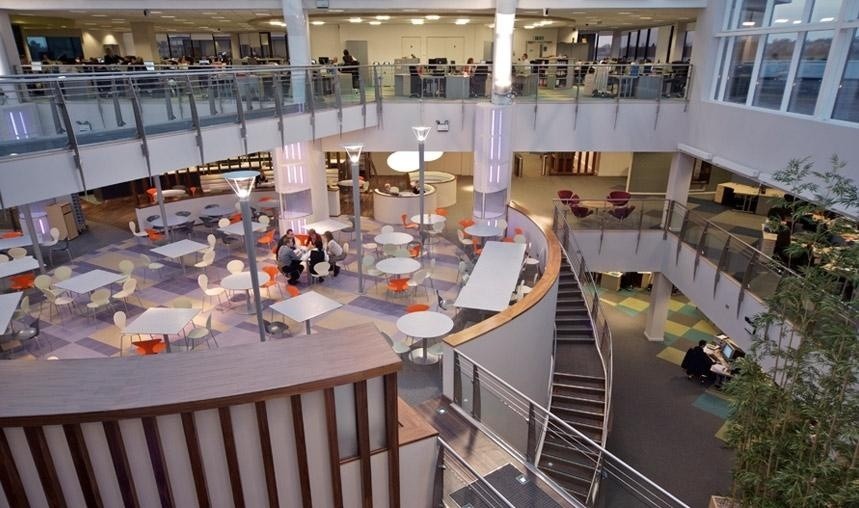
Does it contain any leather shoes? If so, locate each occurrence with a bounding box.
[288,278,297,285]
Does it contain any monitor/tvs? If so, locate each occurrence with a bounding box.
[721,341,745,364]
[319,57,328,64]
[27,35,50,53]
[429,59,436,69]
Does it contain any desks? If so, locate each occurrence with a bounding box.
[702,343,735,390]
[590,271,652,291]
[396,309,454,365]
[61,57,539,101]
[715,182,784,216]
[575,71,690,100]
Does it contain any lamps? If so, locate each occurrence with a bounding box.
[436,120,449,132]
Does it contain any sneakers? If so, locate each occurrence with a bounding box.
[334,266,340,276]
[319,278,324,282]
[328,265,334,270]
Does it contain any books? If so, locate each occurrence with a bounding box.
[534,59,567,88]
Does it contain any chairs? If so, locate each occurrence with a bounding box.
[0,186,544,357]
[681,347,709,385]
[768,194,846,275]
[556,189,637,228]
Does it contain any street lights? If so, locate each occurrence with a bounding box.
[221,170,267,343]
[340,142,367,296]
[411,125,432,245]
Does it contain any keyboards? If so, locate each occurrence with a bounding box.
[709,353,720,362]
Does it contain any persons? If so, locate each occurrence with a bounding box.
[464,58,474,72]
[384,183,391,194]
[277,237,304,284]
[409,180,425,194]
[694,340,707,360]
[306,239,326,283]
[219,54,230,65]
[342,49,353,65]
[103,48,147,71]
[182,56,194,65]
[74,57,83,65]
[305,229,322,250]
[275,229,296,261]
[643,59,654,72]
[521,53,532,73]
[323,230,346,277]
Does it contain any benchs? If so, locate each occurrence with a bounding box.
[408,171,457,207]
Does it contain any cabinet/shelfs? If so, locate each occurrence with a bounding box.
[530,56,576,89]
